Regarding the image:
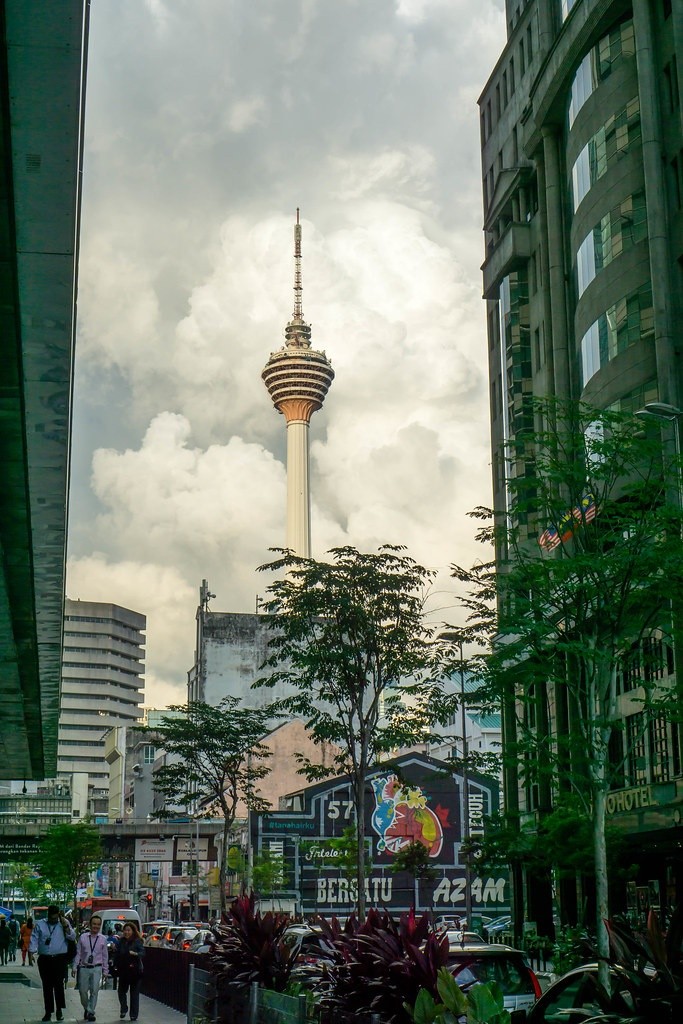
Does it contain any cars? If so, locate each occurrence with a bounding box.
[140,918,242,956]
[525,960,683,1024]
[404,913,541,1024]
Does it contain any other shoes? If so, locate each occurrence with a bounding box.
[88,1013,96,1021]
[13,955,15,961]
[131,1017,136,1021]
[121,1012,126,1018]
[29,963,34,966]
[9,959,12,961]
[56,1011,64,1020]
[42,1013,51,1021]
[22,963,25,966]
[84,1009,89,1019]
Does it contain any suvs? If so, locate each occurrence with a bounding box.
[277,922,345,993]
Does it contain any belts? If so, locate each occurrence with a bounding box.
[82,964,102,968]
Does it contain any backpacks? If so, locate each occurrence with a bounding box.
[8,920,17,937]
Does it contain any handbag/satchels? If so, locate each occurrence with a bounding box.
[18,939,24,948]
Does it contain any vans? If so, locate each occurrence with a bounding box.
[90,908,142,944]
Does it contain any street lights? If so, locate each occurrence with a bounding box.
[435,632,473,931]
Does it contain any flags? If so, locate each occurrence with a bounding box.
[537,483,605,555]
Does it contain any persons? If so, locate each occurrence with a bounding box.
[0,905,145,1020]
